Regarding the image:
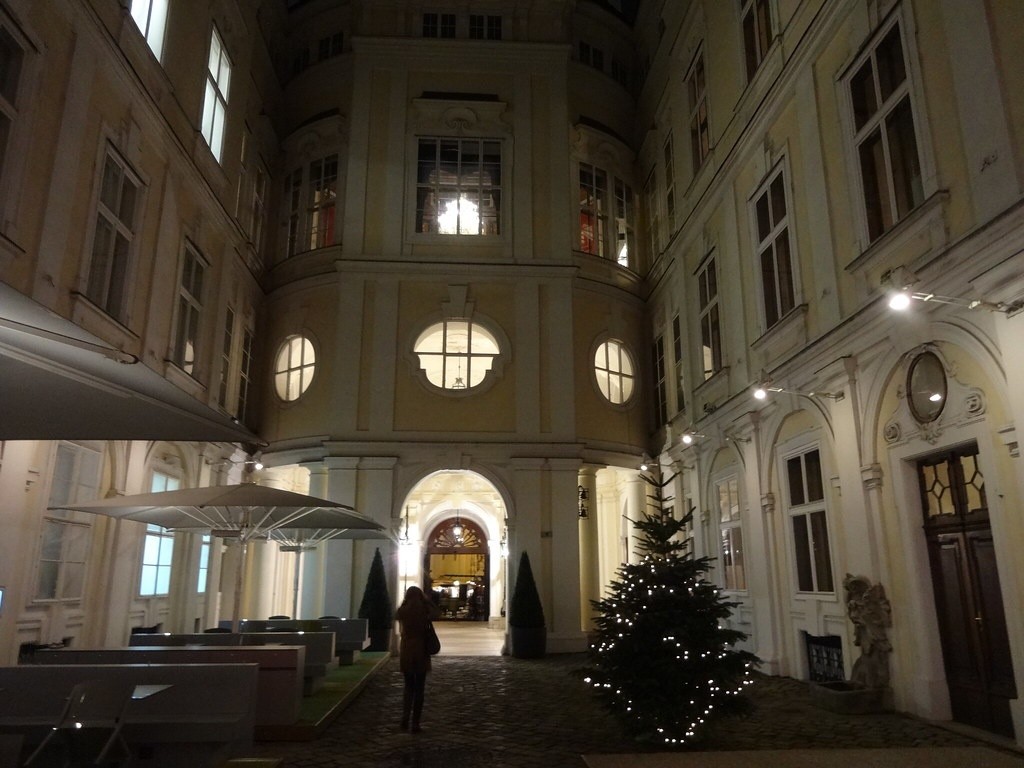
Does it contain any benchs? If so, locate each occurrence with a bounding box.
[17,645,306,725]
[128,632,340,697]
[0,663,261,768]
[218,618,371,664]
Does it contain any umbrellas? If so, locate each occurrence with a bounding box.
[167,527,392,619]
[58,482,353,633]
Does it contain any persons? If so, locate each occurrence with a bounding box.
[394,586,440,732]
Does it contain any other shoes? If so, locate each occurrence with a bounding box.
[399,722,410,734]
[411,721,427,734]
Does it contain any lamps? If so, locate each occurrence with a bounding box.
[888,285,1024,319]
[206,459,263,470]
[640,463,695,472]
[754,387,845,403]
[681,434,752,445]
[452,509,463,539]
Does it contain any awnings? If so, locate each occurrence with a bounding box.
[0,279,271,446]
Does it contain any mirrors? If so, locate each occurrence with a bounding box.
[906,351,947,424]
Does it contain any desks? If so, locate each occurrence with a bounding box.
[16,681,175,767]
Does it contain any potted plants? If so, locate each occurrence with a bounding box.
[358,547,394,651]
[508,550,547,661]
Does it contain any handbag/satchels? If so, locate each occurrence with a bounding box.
[427,622,441,656]
